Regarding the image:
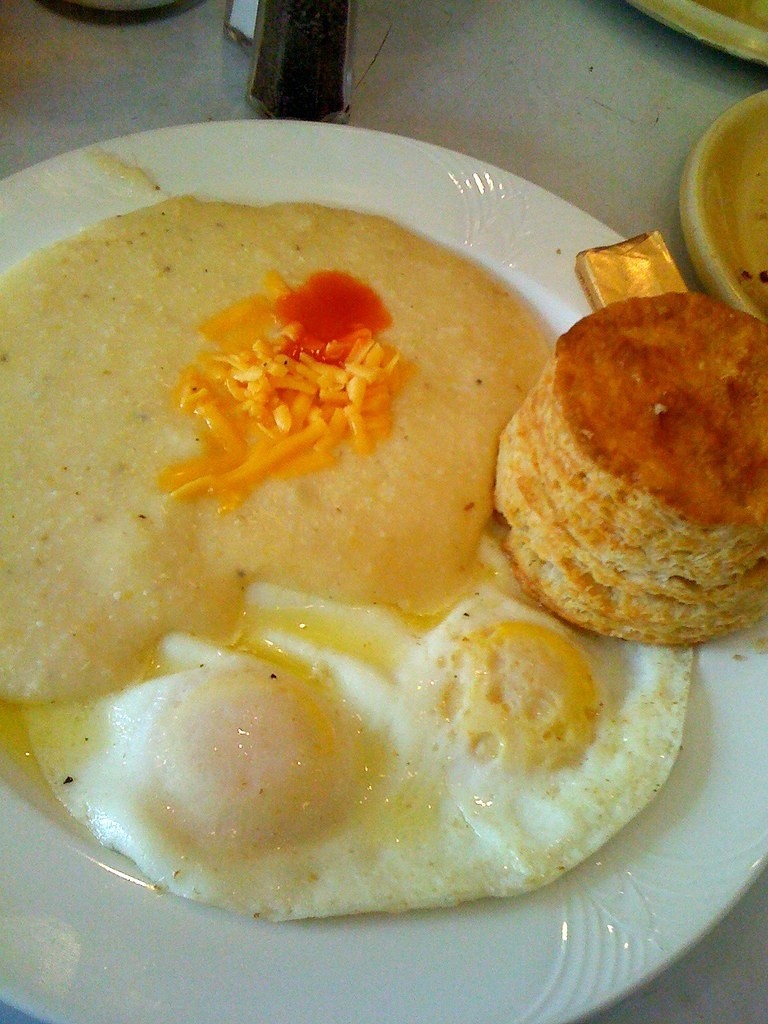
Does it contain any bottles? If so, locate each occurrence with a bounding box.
[247,1,353,124]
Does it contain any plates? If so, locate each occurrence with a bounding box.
[625,0,768,67]
[0,121,768,1024]
[680,89,768,330]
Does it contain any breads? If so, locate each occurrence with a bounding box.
[492,292,768,646]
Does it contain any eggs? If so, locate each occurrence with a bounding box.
[23,525,695,922]
